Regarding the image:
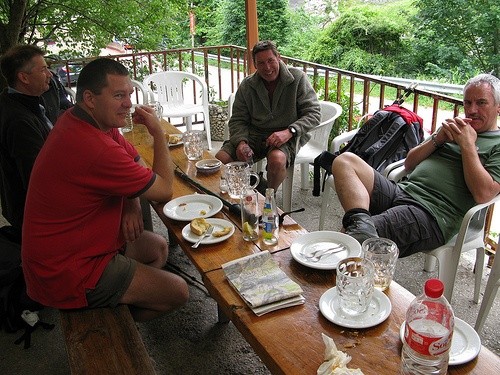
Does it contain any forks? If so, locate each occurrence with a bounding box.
[191,225,214,248]
[299,242,346,261]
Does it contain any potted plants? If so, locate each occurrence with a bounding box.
[200,85,228,141]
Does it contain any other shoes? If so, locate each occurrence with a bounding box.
[342,212,380,246]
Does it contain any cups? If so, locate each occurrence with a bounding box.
[184,130,204,161]
[362,237,399,292]
[144,100,163,121]
[224,160,260,199]
[241,193,259,242]
[336,257,375,315]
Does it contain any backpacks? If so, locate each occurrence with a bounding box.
[40,69,74,126]
[0,230,54,349]
[249,171,269,198]
[312,103,426,197]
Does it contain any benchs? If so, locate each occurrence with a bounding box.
[59,304,158,375]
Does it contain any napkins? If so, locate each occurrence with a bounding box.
[222,250,306,317]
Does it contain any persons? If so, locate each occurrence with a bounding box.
[332,74,500,264]
[0,44,189,321]
[214,41,321,198]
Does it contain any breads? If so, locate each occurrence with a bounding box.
[190,218,210,235]
[169,136,179,144]
[213,226,231,237]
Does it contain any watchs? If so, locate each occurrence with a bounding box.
[288,126,298,136]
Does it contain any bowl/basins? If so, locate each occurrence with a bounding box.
[196,159,222,173]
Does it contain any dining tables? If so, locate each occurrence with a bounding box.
[119,118,308,275]
[204,242,500,375]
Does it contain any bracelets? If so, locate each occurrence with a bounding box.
[431,133,441,148]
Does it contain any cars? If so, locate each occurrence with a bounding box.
[46,53,83,87]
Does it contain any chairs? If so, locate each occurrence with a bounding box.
[65,71,500,335]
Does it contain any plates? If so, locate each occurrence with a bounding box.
[400,314,482,365]
[319,285,392,328]
[162,194,224,221]
[165,134,186,147]
[291,230,362,271]
[181,217,236,244]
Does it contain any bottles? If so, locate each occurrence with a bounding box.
[261,188,279,245]
[119,108,134,133]
[399,280,455,375]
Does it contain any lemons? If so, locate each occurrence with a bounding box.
[243,222,254,236]
